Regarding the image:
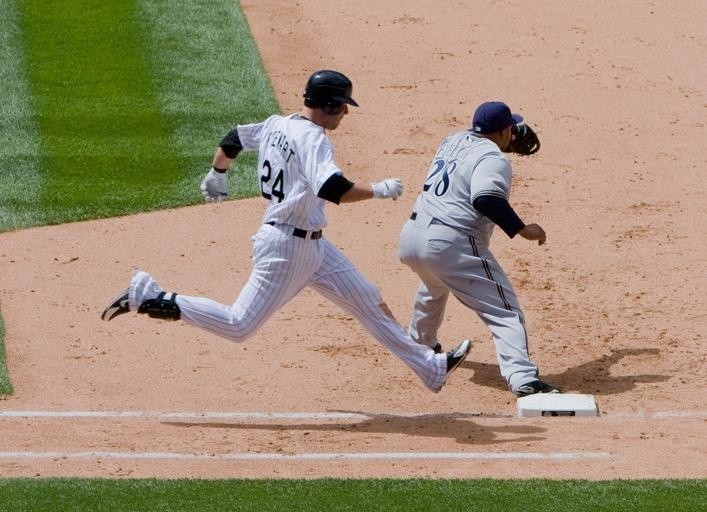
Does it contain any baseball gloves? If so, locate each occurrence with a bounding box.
[503,113,541,156]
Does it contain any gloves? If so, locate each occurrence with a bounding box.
[368,178,404,202]
[200,166,228,204]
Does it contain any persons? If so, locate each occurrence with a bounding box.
[396,101,560,397]
[97,67,474,396]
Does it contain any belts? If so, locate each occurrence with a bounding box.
[410,212,445,226]
[268,222,323,240]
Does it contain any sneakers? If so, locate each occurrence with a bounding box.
[515,380,562,397]
[434,339,473,394]
[101,286,131,323]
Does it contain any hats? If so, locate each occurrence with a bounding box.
[303,69,359,107]
[472,101,524,135]
